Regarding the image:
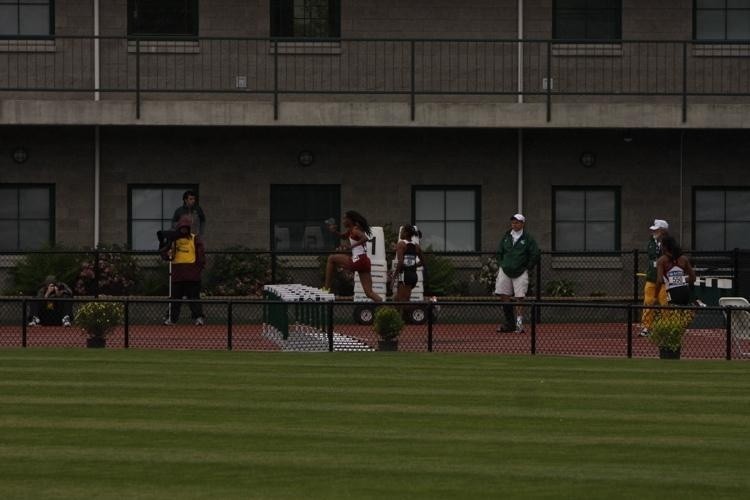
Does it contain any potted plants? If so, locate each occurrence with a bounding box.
[648,309,696,360]
[373,307,404,351]
[74,301,124,348]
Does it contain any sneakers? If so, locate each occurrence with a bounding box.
[29,317,39,326]
[195,316,204,325]
[431,295,440,310]
[62,316,72,326]
[640,329,650,336]
[320,287,331,293]
[164,319,175,325]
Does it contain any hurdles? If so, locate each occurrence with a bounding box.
[262,283,337,353]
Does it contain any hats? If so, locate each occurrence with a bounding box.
[510,214,524,222]
[650,219,669,231]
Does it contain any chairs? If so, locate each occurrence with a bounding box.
[719,298,750,356]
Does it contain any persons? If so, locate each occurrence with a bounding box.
[319,211,382,303]
[391,225,441,313]
[655,233,707,325]
[638,219,670,337]
[495,214,540,333]
[165,214,206,326]
[28,275,73,328]
[172,192,205,237]
